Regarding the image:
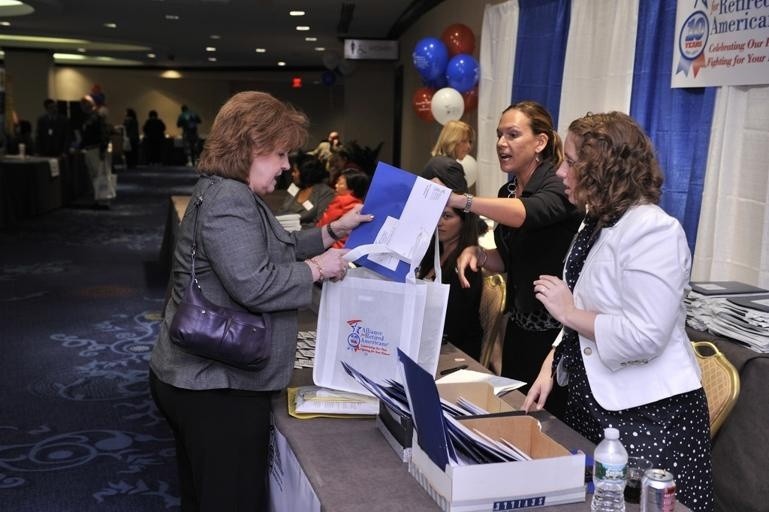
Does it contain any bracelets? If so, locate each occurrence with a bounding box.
[309,258,325,281]
[481,250,489,266]
[464,193,474,213]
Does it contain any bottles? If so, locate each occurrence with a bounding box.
[590,428,629,512]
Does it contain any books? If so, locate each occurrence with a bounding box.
[686,273,769,354]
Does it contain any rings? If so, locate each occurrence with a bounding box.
[541,287,547,292]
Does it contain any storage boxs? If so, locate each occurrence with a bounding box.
[375,376,519,459]
[403,414,587,512]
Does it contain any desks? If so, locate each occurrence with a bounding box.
[170,190,698,511]
[676,320,768,511]
[0,155,66,220]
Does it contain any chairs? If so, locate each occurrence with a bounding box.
[472,266,507,367]
[690,334,745,445]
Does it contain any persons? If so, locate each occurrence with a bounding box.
[521,107,716,512]
[421,121,470,195]
[0,83,202,210]
[417,202,484,363]
[141,90,377,510]
[432,98,585,421]
[278,130,370,249]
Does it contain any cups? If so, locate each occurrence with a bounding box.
[624,455,653,506]
[19,144,26,157]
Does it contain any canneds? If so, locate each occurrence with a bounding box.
[640,468,676,512]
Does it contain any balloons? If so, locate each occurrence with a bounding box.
[430,86,465,127]
[441,23,475,57]
[445,53,480,95]
[461,86,480,116]
[410,37,449,83]
[412,87,436,125]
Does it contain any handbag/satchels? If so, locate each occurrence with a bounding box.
[168,276,273,373]
[93,173,117,201]
[310,264,453,398]
